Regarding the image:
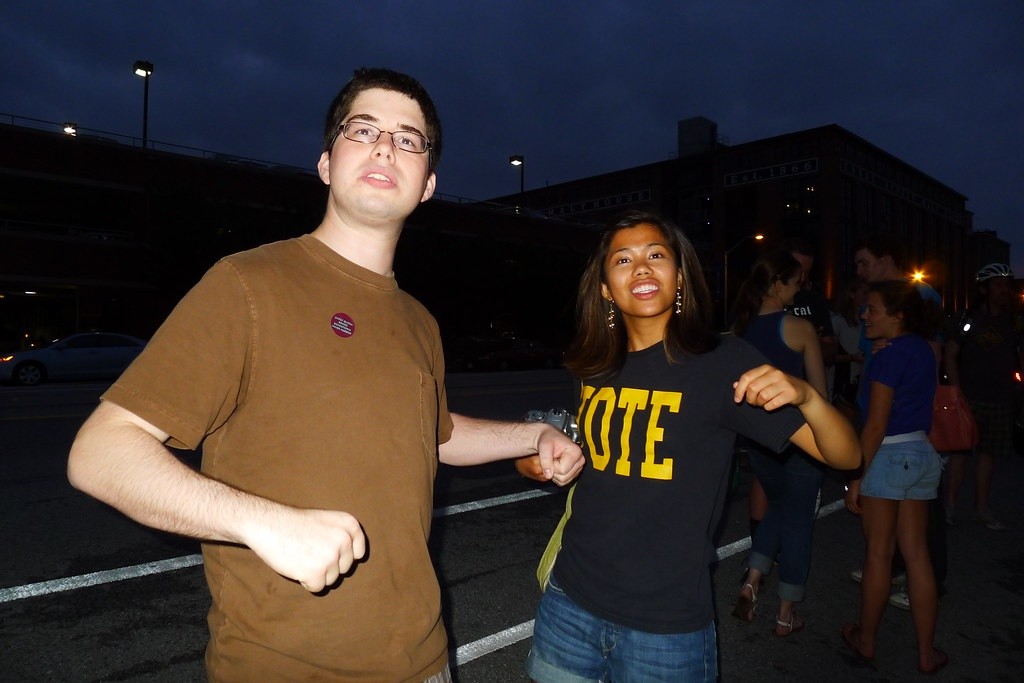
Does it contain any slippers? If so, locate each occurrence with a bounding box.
[917,648,950,674]
[840,623,874,661]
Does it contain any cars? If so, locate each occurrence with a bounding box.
[0,332,149,385]
[496,205,573,224]
[489,337,568,371]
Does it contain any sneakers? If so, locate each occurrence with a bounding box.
[886,575,911,611]
[850,568,863,584]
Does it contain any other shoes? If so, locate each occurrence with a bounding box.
[945,507,958,524]
[974,509,1005,531]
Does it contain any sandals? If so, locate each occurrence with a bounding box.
[772,611,805,637]
[731,584,757,621]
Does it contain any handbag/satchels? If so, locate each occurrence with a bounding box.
[926,345,978,451]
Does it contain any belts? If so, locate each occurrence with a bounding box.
[880,431,927,444]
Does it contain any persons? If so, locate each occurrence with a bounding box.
[65,69,584,682]
[729,253,828,637]
[515,204,861,683]
[750,231,833,548]
[944,262,1019,530]
[851,233,947,610]
[848,273,868,304]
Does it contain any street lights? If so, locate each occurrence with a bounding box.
[509,155,525,207]
[721,233,764,333]
[132,60,155,147]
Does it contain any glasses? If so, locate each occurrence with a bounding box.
[329,121,432,173]
